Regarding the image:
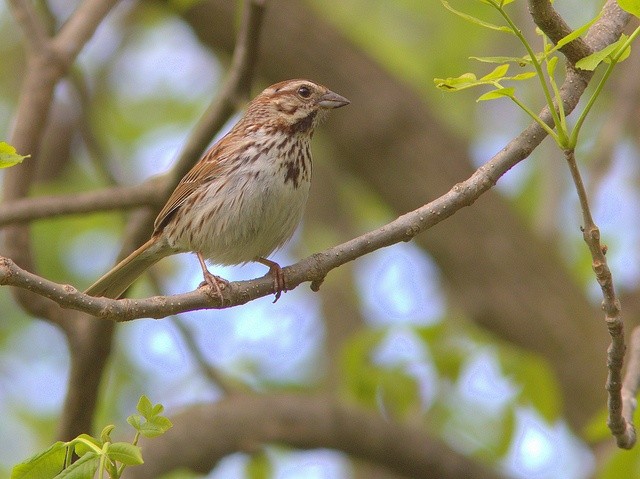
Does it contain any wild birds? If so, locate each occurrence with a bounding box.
[82,78,352,308]
